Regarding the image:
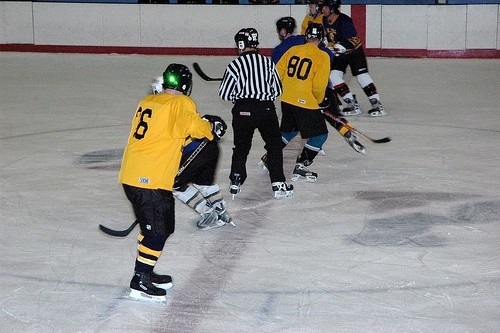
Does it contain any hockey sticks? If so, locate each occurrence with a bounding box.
[329,48,340,51]
[99,124,221,237]
[321,109,390,144]
[193,62,223,81]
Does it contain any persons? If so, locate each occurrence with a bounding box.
[271,17,365,156]
[259,23,330,182]
[117,64,227,300]
[151,76,236,230]
[320,0,387,116]
[218,28,294,201]
[302,0,327,36]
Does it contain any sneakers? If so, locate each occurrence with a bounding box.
[128,272,173,306]
[291,164,318,183]
[195,204,237,231]
[257,154,271,175]
[367,105,388,117]
[339,104,362,116]
[271,182,295,200]
[229,181,242,201]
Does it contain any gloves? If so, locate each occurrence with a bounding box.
[201,114,227,141]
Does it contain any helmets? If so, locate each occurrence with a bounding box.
[306,0,342,12]
[151,76,164,97]
[234,27,259,50]
[161,63,193,97]
[305,22,324,41]
[276,17,296,34]
